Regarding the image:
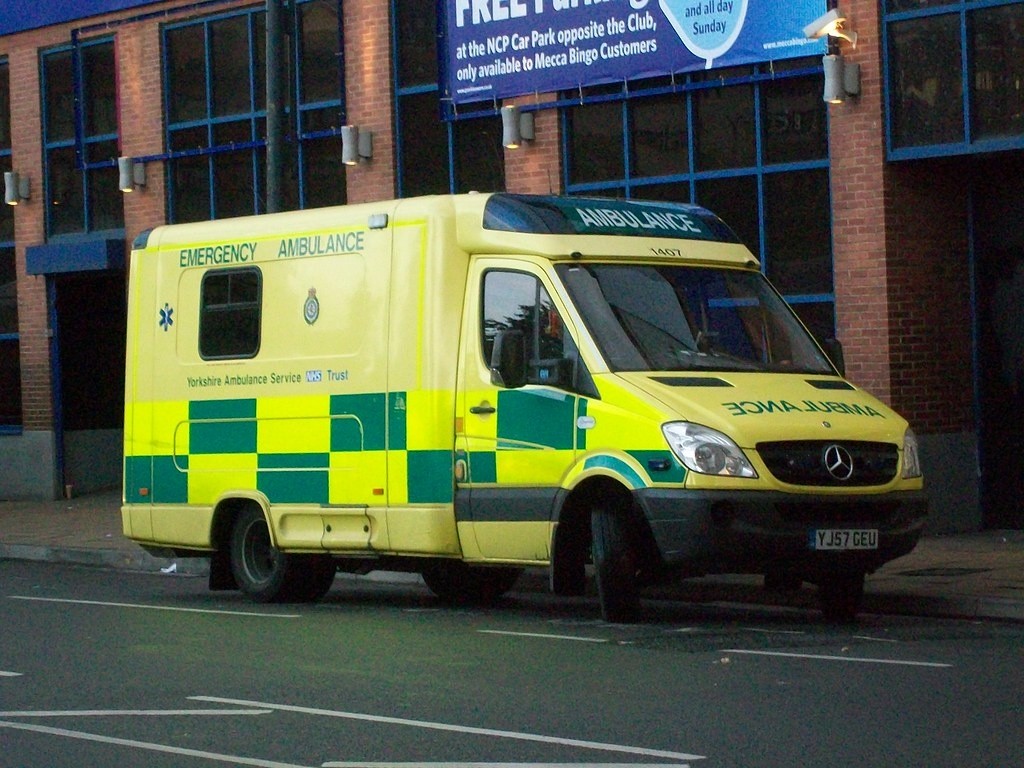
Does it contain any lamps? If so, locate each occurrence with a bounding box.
[822,55,861,103]
[4,172,30,205]
[118,157,145,193]
[501,105,534,148]
[341,125,372,166]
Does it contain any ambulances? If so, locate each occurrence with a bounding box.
[121,189,927,621]
